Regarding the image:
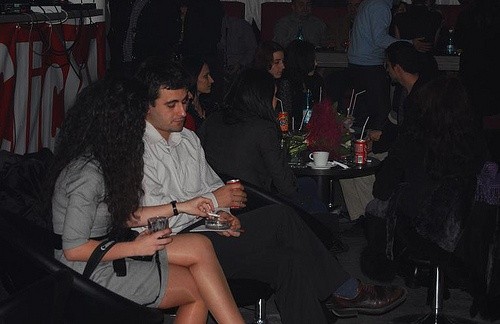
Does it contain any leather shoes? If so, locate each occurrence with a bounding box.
[331,278,409,318]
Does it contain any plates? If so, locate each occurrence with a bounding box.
[307,162,337,169]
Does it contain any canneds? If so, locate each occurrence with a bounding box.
[278,111,288,131]
[226,179,244,209]
[354,139,367,165]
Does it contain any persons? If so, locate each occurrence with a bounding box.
[51,76,245,324]
[455,0,500,114]
[180,54,215,132]
[361,42,440,281]
[134,56,408,324]
[272,0,327,48]
[255,40,321,129]
[347,0,451,154]
[197,68,350,254]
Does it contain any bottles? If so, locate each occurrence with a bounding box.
[446,21,454,54]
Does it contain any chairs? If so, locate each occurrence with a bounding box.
[0,209,164,324]
[388,176,479,324]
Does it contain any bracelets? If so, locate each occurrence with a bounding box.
[171,201,178,215]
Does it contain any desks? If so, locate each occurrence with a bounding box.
[291,153,382,213]
[312,44,464,77]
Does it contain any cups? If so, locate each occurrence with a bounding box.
[148,216,169,240]
[309,152,329,167]
